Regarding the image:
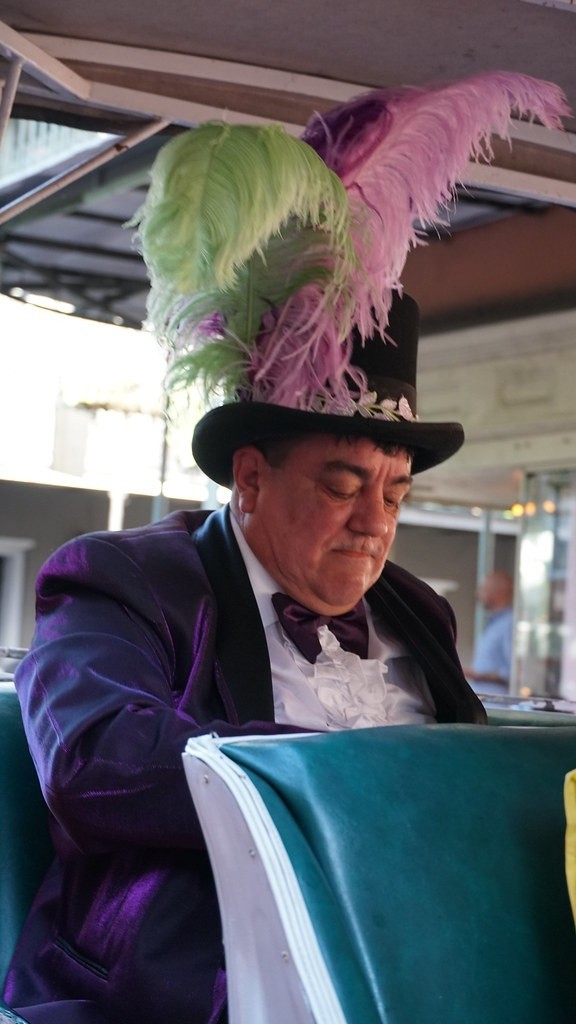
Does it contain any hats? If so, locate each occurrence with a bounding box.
[119,73,575,489]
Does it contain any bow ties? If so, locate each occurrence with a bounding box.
[270,591,371,664]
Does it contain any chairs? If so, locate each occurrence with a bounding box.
[0,690,576,1007]
[182,725,576,1024]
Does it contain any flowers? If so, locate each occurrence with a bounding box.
[311,389,421,423]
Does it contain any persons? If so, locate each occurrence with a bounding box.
[0,289,490,1024]
[456,572,514,710]
